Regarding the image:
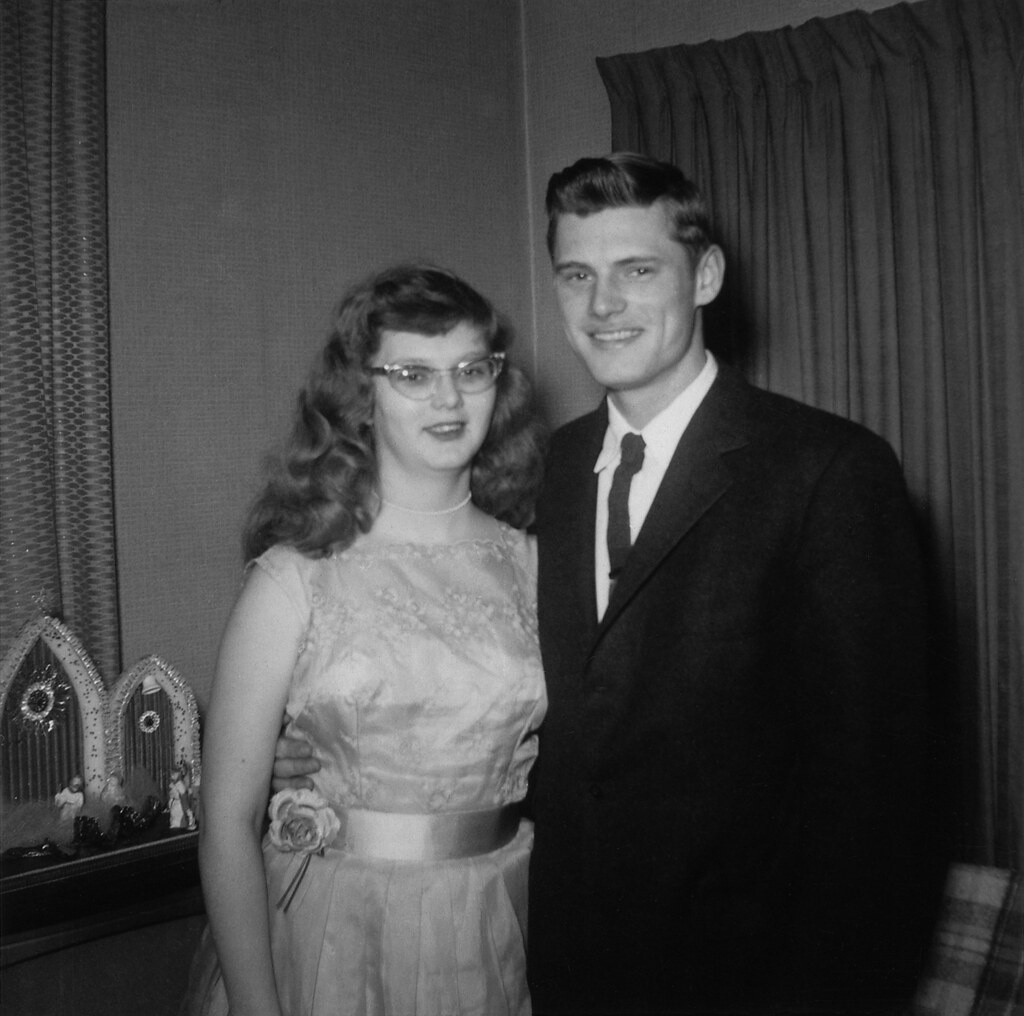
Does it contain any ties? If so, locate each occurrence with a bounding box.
[606,432,647,600]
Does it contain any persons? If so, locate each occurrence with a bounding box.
[197,265,547,1016]
[272,153,944,1016]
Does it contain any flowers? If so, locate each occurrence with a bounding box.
[269,786,341,857]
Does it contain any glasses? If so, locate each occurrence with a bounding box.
[365,350,508,401]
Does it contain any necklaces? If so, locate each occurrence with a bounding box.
[371,488,471,517]
